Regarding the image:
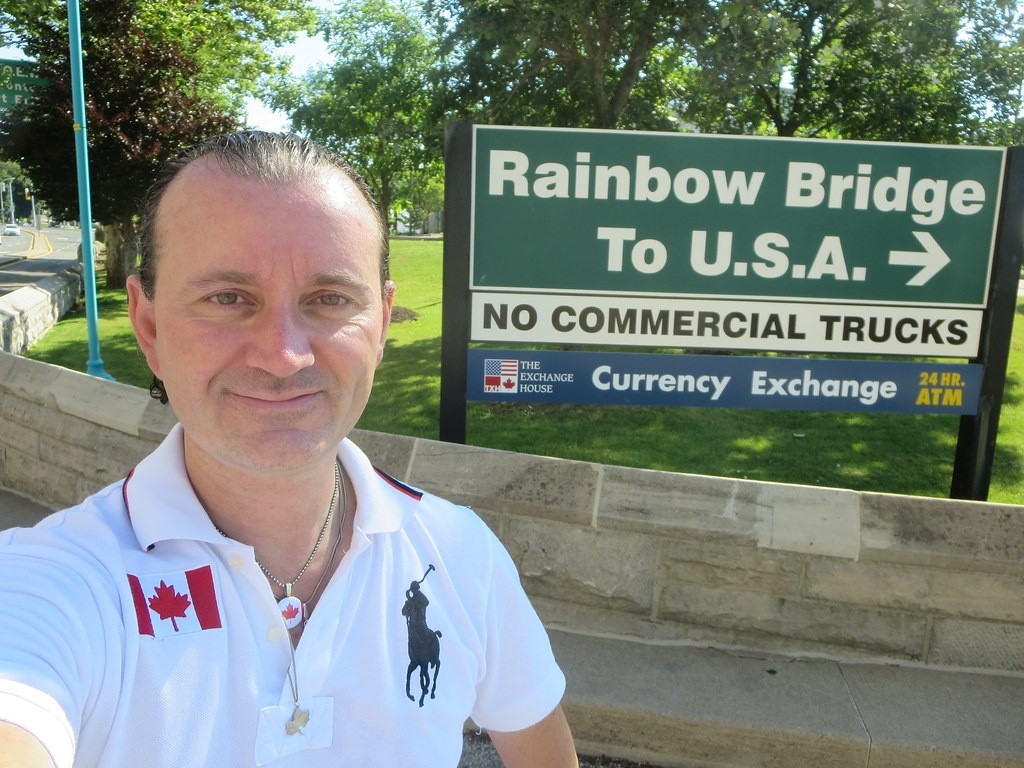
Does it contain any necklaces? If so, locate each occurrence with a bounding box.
[213,461,346,734]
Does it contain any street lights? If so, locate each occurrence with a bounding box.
[0,177,15,224]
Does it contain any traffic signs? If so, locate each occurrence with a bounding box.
[467,122,1009,309]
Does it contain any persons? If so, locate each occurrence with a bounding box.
[0,131,581,768]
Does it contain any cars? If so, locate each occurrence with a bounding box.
[3,224,21,235]
